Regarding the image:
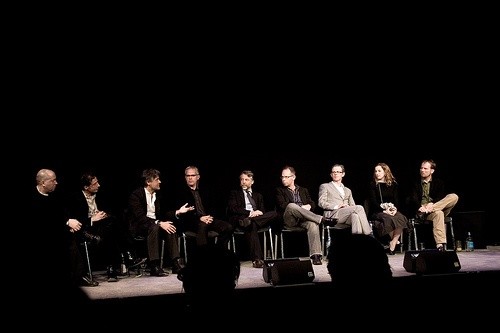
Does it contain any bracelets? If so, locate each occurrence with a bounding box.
[157,221,162,225]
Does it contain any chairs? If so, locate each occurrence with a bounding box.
[78,202,456,284]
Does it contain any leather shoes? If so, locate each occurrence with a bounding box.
[128,256,147,268]
[173,259,186,272]
[107,267,118,281]
[81,275,99,287]
[149,266,169,276]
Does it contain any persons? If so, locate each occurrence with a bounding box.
[13,168,102,287]
[228,168,278,268]
[181,261,250,333]
[274,168,338,264]
[317,163,373,236]
[370,163,410,255]
[126,167,195,278]
[167,166,233,271]
[408,159,459,252]
[70,173,141,281]
[0,233,500,333]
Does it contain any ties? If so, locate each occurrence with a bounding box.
[246,191,257,210]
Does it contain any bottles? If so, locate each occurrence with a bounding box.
[466,232,474,252]
[266,249,271,259]
[120,252,126,274]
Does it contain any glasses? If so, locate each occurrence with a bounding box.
[185,174,198,176]
[330,171,343,175]
[280,175,294,179]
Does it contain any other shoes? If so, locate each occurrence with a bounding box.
[416,211,428,221]
[252,259,265,267]
[396,242,401,251]
[389,247,395,254]
[437,246,445,252]
[312,255,323,265]
[323,217,338,227]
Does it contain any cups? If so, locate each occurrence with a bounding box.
[456,240,462,252]
